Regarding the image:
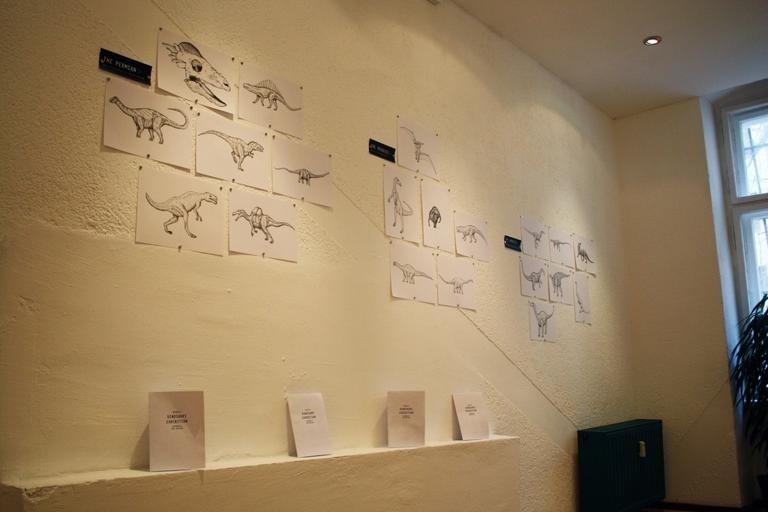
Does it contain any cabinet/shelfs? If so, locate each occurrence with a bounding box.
[577,416,667,512]
